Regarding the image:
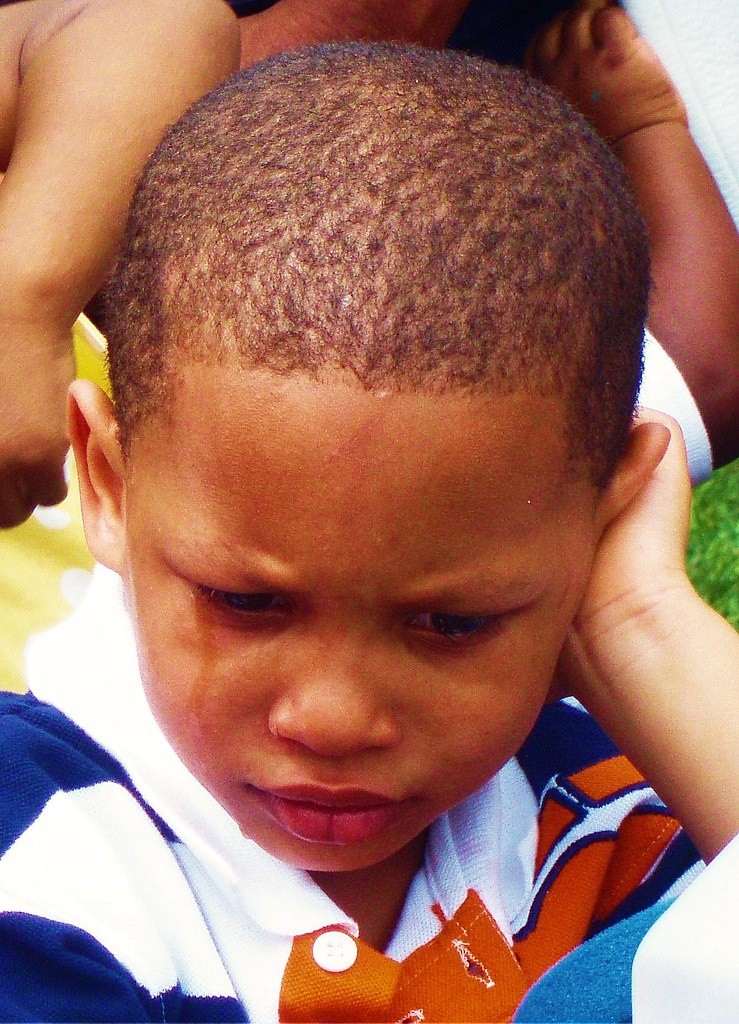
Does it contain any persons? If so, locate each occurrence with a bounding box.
[0,0,739,1024]
